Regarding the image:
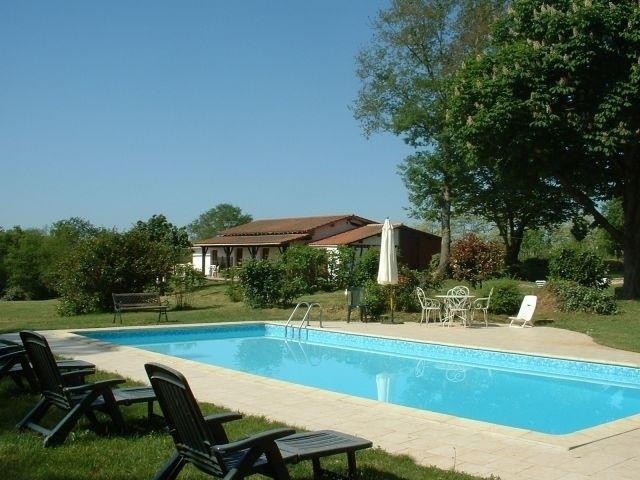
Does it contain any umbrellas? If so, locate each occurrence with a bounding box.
[374,216,401,323]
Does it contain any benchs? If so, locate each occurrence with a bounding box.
[111,291,170,324]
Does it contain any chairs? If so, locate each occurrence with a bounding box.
[345,285,367,324]
[144,362,371,478]
[15,331,168,449]
[507,295,538,328]
[1,338,95,392]
[416,285,494,328]
